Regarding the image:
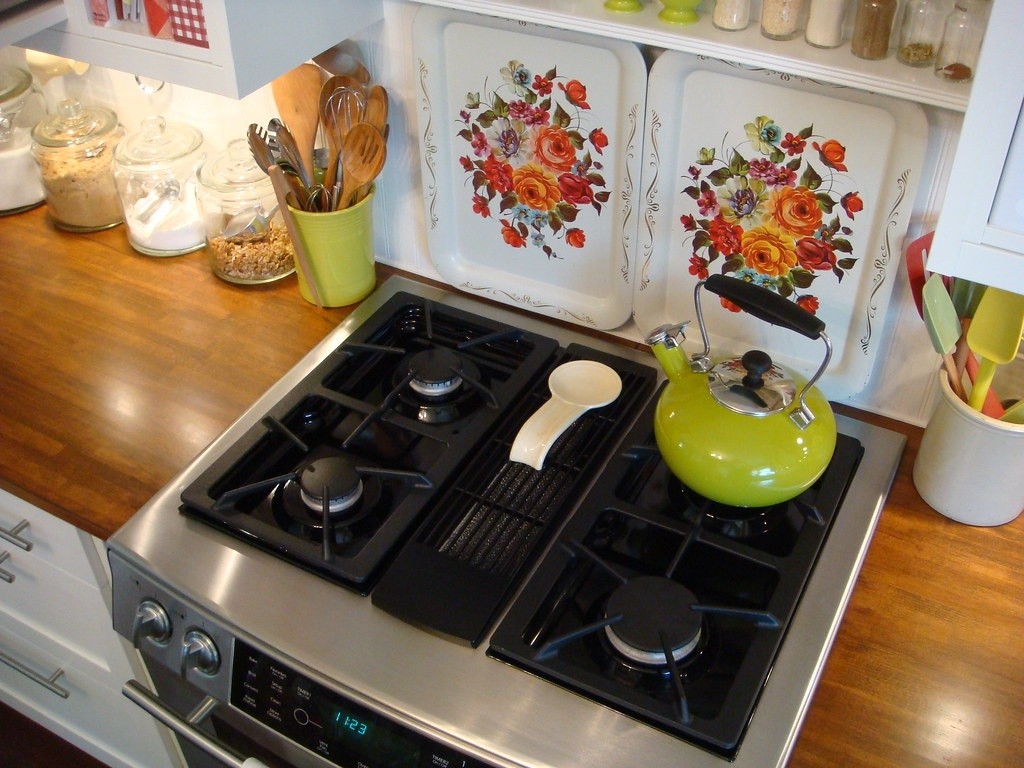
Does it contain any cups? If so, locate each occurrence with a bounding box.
[285,170,376,308]
[913,353,1024,526]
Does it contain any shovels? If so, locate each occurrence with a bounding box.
[903,231,1024,432]
[270,62,324,185]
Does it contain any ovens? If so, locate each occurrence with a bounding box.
[122,648,333,768]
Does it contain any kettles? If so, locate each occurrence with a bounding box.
[646,275,837,507]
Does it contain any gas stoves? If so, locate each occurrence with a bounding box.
[108,270,903,768]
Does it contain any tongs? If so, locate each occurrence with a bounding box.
[247,118,313,191]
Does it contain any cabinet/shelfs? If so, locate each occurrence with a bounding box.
[0,199,387,768]
[0,0,382,98]
[923,0,1024,299]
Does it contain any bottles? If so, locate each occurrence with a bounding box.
[0,65,49,216]
[31,99,136,233]
[195,138,295,283]
[112,118,225,257]
[604,0,987,83]
